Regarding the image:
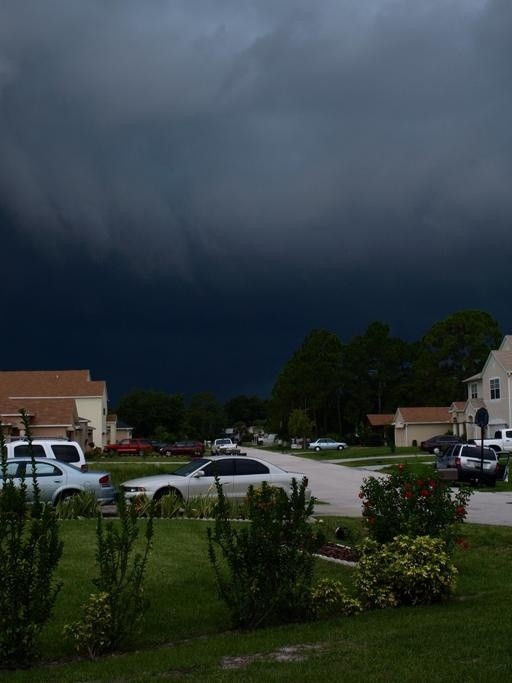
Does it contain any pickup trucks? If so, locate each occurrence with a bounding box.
[468,429,512,454]
[211,438,238,455]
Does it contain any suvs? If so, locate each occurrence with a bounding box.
[435,443,510,489]
[104,438,205,457]
[4,435,88,472]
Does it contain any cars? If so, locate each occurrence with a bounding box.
[0,457,116,518]
[421,434,468,454]
[307,438,348,452]
[119,455,311,505]
